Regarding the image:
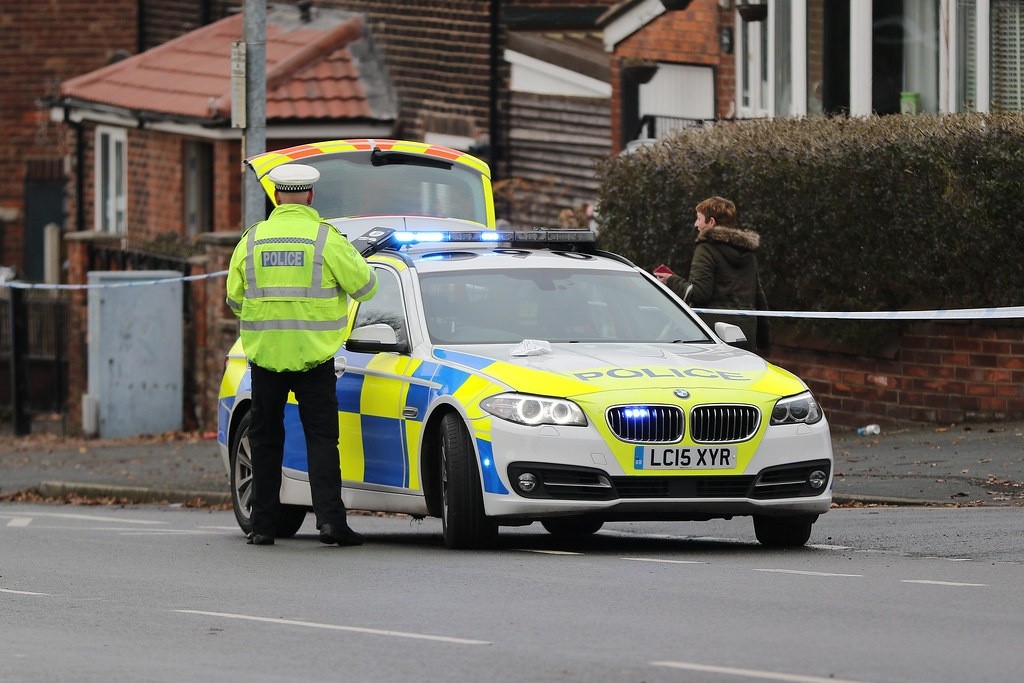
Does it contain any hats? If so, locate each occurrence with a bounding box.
[268,165,320,192]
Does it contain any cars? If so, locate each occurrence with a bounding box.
[219,139,836,553]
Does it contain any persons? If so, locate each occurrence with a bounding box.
[224,162,379,548]
[656,199,774,359]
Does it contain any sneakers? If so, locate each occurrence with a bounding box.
[245,531,274,544]
[318,524,367,545]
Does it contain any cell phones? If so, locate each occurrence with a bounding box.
[653,264,675,275]
